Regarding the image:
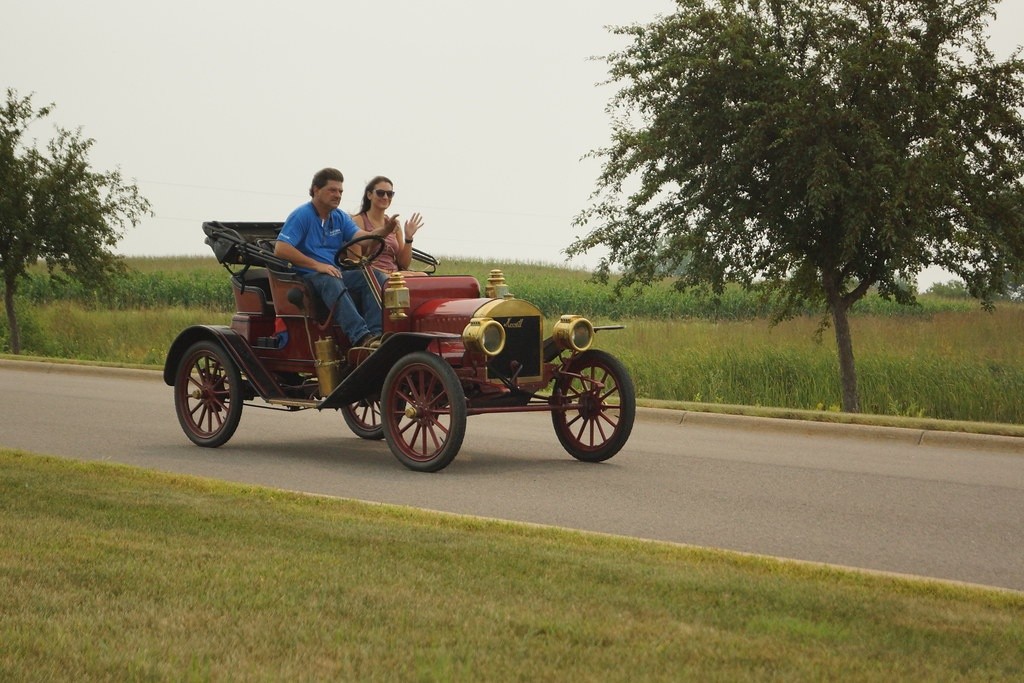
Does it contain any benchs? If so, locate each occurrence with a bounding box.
[256,241,328,318]
[231,268,274,314]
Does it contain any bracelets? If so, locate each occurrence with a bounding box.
[405,239,413,243]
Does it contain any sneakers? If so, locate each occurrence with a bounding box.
[363,331,396,354]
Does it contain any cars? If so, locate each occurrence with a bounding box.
[162,221,636,474]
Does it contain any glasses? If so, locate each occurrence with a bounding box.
[372,188,395,199]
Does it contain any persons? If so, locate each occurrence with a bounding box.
[274,168,399,347]
[346,176,427,276]
